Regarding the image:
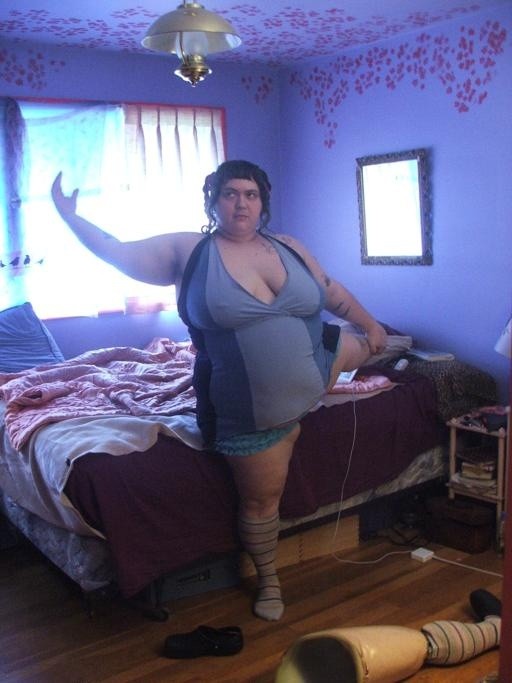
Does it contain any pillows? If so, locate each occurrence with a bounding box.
[0,301,66,374]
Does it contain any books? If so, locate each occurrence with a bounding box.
[443,444,498,498]
[406,348,454,362]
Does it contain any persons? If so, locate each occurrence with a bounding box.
[51,160,390,622]
[272,586,502,683]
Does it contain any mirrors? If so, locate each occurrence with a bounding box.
[354,149,432,266]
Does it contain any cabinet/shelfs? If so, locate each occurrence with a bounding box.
[445,410,507,553]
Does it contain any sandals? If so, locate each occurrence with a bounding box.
[164,625,243,659]
[470,589,501,618]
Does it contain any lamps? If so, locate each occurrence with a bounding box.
[140,1,242,88]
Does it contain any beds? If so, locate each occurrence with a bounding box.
[2,350,463,603]
